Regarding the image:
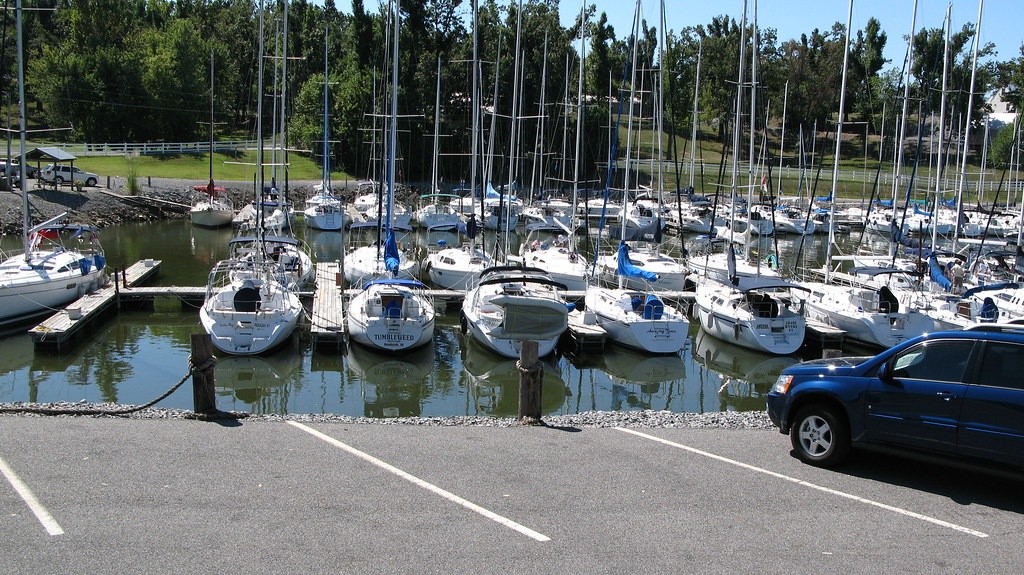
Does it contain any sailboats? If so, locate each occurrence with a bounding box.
[188,330,806,416]
[0,1,110,339]
[197,0,1024,357]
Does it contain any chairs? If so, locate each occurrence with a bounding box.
[0,181,1024,325]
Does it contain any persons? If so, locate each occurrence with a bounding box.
[975,257,988,287]
[950,259,965,295]
[761,174,767,192]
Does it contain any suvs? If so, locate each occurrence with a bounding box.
[40,164,100,188]
[766,318,1024,488]
[0,157,39,179]
